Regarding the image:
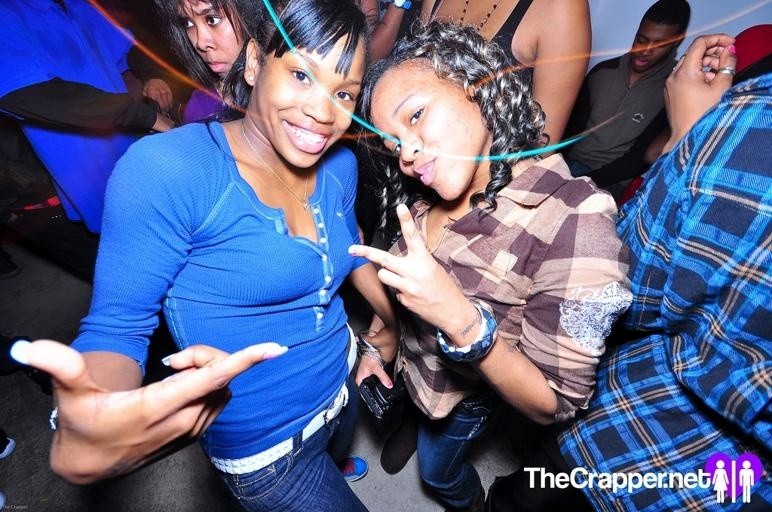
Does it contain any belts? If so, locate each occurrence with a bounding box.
[211,323,358,475]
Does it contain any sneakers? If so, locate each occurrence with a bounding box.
[342,453,368,482]
[382,424,417,475]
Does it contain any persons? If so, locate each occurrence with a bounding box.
[1,1,771,511]
